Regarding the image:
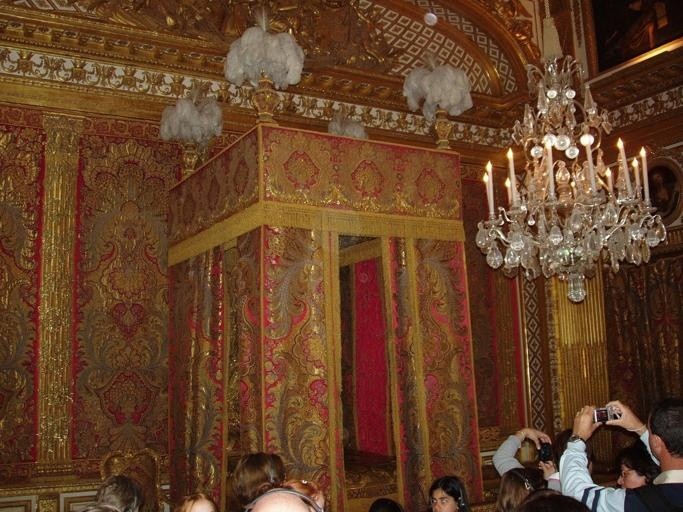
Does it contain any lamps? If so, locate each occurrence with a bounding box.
[474,1,667,303]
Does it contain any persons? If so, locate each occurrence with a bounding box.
[491,399,682,512]
[428,475,471,512]
[86,452,325,512]
[647,170,673,208]
[369,497,404,512]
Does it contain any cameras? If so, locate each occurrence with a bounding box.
[594,408,614,422]
[539,442,553,461]
